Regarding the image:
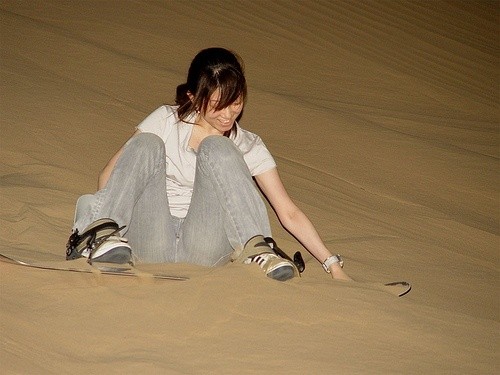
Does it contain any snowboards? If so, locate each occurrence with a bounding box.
[1,244,411,297]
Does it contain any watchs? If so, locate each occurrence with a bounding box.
[322,254,344,274]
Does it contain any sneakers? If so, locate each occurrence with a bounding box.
[75,218,132,263]
[238,234,299,280]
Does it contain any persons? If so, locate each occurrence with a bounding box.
[66,47,354,282]
